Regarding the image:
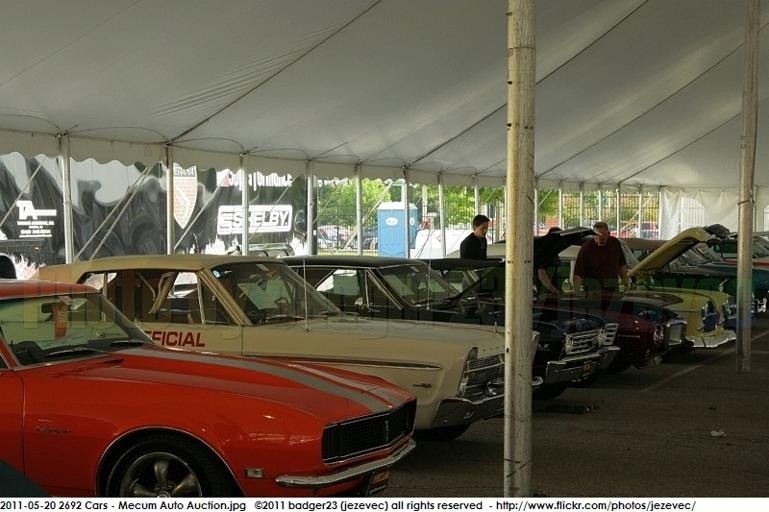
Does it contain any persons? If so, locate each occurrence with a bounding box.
[459,214,489,297]
[536,228,568,294]
[573,223,629,306]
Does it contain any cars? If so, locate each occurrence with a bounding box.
[277,255,609,400]
[0,277,418,495]
[34,252,542,444]
